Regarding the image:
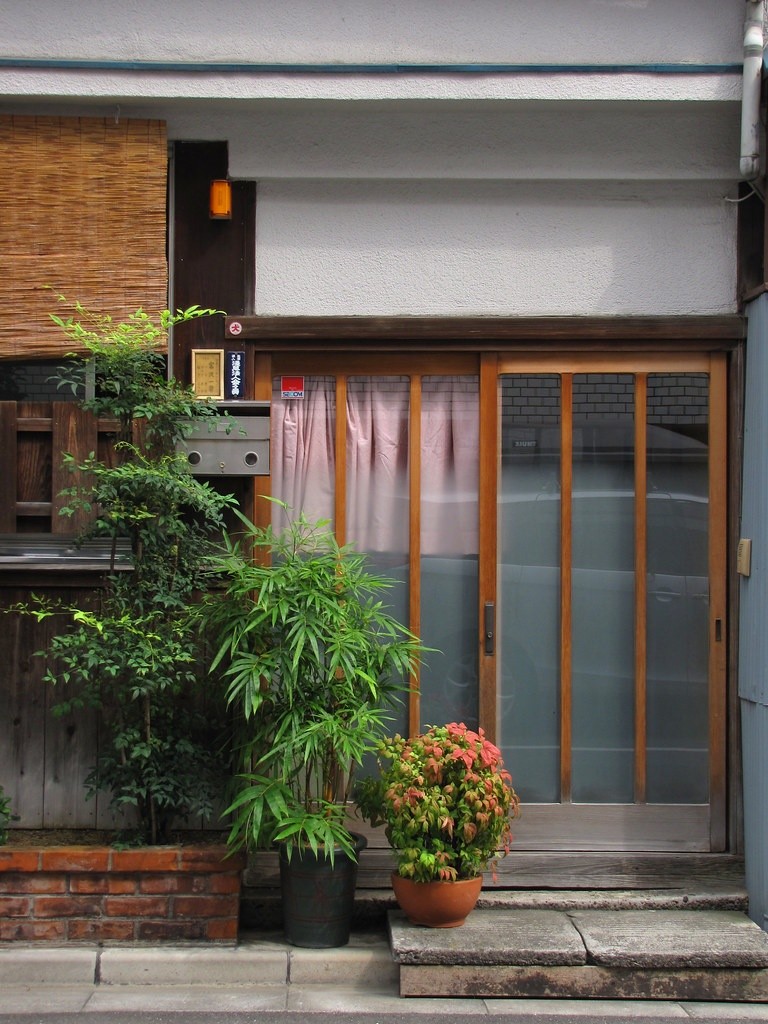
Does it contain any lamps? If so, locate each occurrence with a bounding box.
[208,179,233,220]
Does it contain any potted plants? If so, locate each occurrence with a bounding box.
[353,722,521,930]
[177,495,444,949]
